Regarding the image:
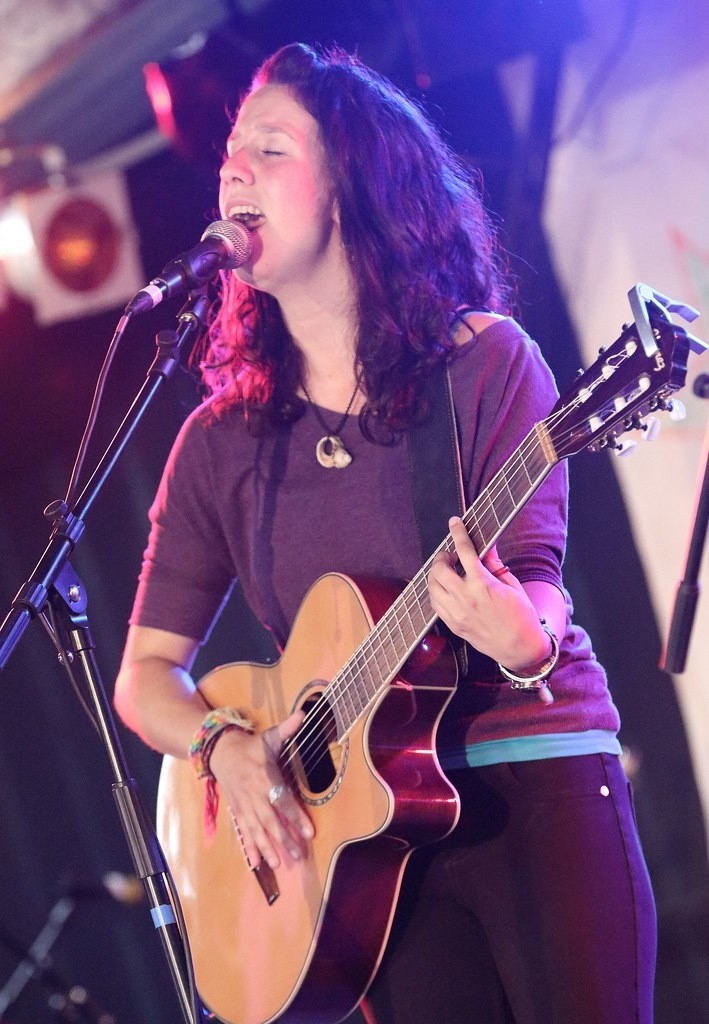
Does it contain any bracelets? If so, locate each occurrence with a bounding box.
[498,616,561,704]
[187,706,256,842]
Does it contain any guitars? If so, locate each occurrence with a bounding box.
[155,280,708,1024]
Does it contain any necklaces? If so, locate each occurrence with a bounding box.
[290,361,367,468]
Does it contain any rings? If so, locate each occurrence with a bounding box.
[268,784,294,804]
[491,566,509,578]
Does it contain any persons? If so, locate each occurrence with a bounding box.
[113,41,659,1024]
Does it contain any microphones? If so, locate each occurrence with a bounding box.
[126,221,253,319]
